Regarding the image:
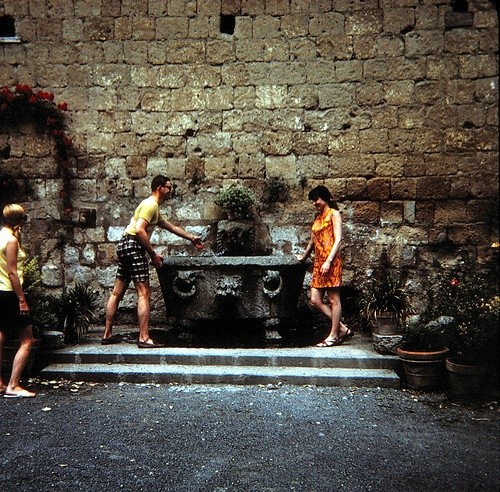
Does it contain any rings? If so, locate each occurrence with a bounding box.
[23,313,25,314]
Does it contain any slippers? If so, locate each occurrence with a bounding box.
[4,390,36,397]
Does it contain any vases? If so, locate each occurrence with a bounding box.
[397,347,449,391]
[446,357,488,396]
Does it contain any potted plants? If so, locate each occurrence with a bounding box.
[357,248,417,335]
[1,255,100,379]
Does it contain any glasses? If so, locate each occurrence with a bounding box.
[163,184,171,191]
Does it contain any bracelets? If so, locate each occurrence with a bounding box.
[326,258,333,262]
[149,251,155,256]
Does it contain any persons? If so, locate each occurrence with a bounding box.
[100,174,206,348]
[0,204,38,399]
[294,185,354,347]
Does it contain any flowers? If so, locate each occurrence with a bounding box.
[398,242,500,364]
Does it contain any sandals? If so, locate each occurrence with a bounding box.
[101,335,122,345]
[316,328,354,347]
[138,337,164,348]
